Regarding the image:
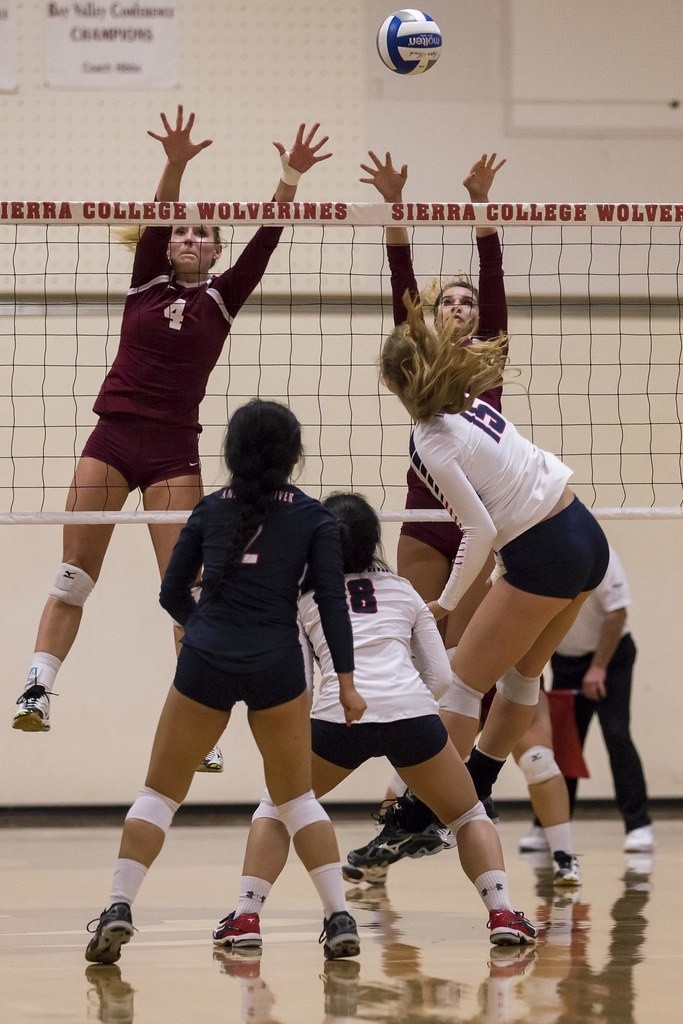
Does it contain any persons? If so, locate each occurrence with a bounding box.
[212,301,657,959]
[82,397,360,964]
[12,104,333,774]
[360,150,510,823]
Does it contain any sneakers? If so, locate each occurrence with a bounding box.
[347,807,443,869]
[195,746,224,773]
[550,850,583,887]
[487,908,537,945]
[342,863,388,884]
[435,797,500,849]
[12,668,59,732]
[212,910,262,947]
[318,911,360,958]
[518,826,550,852]
[624,824,653,855]
[85,902,139,963]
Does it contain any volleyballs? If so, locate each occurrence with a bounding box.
[376,8,445,77]
